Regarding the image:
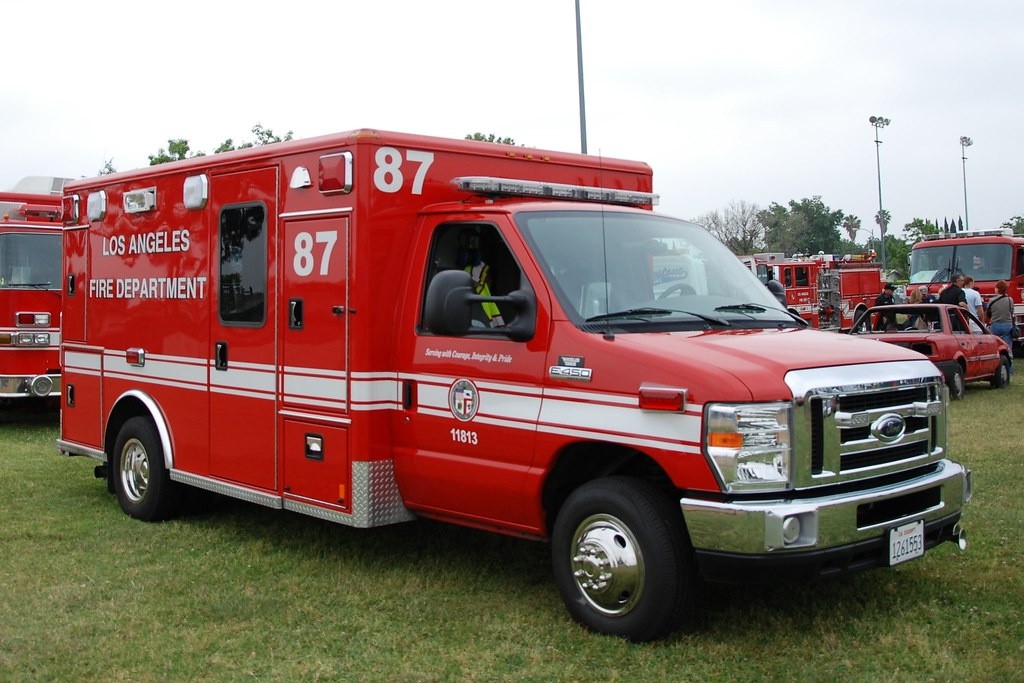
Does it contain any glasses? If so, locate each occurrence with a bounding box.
[962,280,963,281]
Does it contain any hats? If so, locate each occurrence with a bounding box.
[918,285,928,294]
[884,283,897,290]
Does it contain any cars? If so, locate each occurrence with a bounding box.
[848,304,1012,398]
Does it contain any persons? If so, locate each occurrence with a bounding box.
[919,285,936,303]
[961,277,984,334]
[986,280,1017,375]
[873,285,897,329]
[908,288,926,328]
[939,274,968,331]
[938,284,948,300]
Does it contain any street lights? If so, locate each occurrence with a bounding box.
[869,117,893,290]
[958,134,973,229]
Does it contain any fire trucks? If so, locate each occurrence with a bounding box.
[0,191,63,398]
[56,128,972,638]
[905,228,1024,346]
[745,250,882,334]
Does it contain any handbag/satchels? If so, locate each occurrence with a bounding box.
[987,295,1007,317]
[1010,327,1021,339]
